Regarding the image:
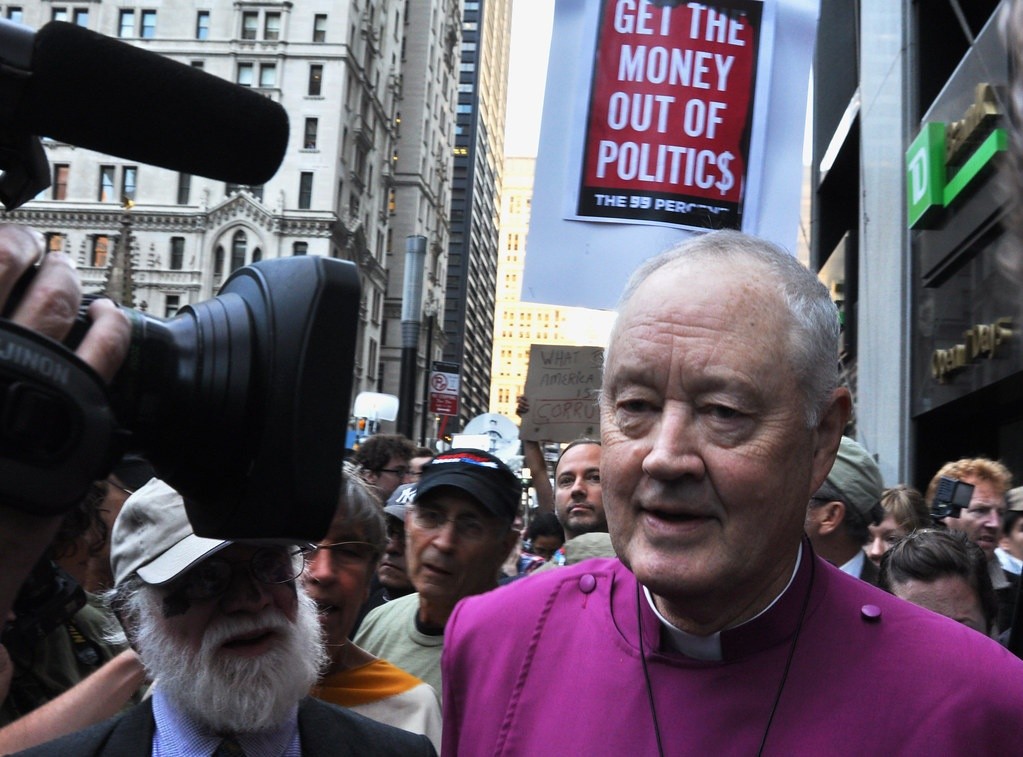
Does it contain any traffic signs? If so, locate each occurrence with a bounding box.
[428,370,460,395]
[429,392,458,416]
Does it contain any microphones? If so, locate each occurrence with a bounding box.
[0,19,289,187]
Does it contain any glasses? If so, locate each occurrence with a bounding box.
[376,468,413,479]
[410,505,514,539]
[122,544,321,602]
[318,542,383,564]
[384,526,408,547]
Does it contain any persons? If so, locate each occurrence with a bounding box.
[862,486,931,566]
[0,221,151,757]
[352,447,522,708]
[441,228,1023,757]
[294,474,443,757]
[496,396,618,586]
[923,459,1023,661]
[350,434,435,640]
[876,529,997,638]
[804,435,885,586]
[0,478,437,757]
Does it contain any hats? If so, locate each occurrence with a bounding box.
[379,483,417,522]
[562,531,623,567]
[414,448,522,531]
[824,434,881,525]
[110,476,315,586]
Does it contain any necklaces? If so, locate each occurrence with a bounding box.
[637,530,815,757]
